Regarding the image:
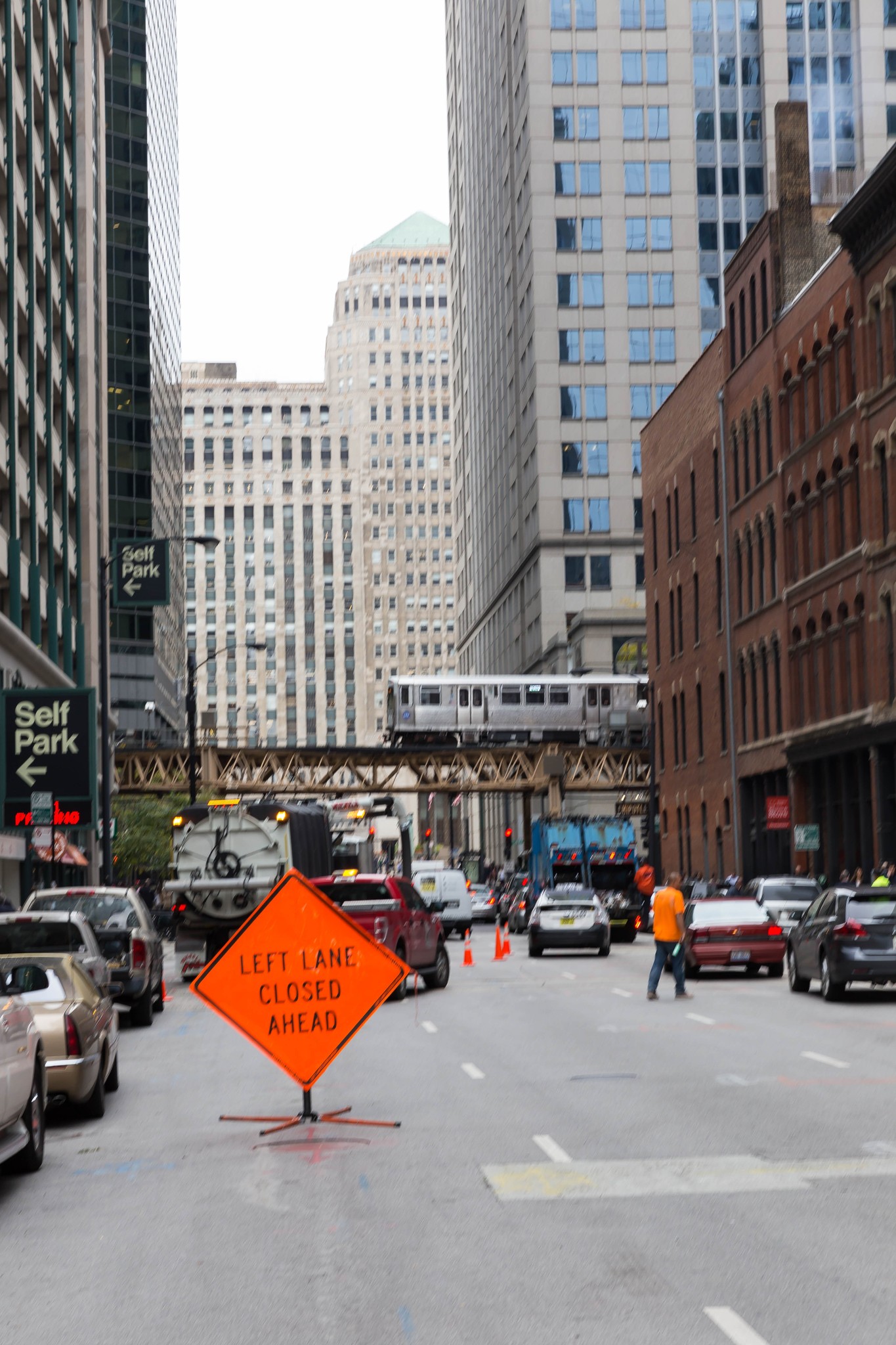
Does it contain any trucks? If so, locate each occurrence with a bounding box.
[527,812,642,945]
[158,796,412,984]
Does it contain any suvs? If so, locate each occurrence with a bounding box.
[15,883,165,1029]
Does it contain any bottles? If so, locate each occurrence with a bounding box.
[672,942,682,957]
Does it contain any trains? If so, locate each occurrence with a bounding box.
[378,671,651,749]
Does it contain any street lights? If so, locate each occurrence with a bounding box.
[96,533,222,885]
[184,642,269,802]
[568,666,656,884]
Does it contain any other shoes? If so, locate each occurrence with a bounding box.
[675,992,692,1000]
[647,991,656,1000]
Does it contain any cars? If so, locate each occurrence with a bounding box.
[742,875,825,949]
[527,879,614,958]
[411,859,542,942]
[0,908,124,1000]
[660,893,789,981]
[0,949,125,1120]
[784,882,896,1001]
[307,868,450,1000]
[0,969,47,1180]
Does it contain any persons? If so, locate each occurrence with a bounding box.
[646,872,693,1000]
[483,857,896,933]
[133,876,176,942]
